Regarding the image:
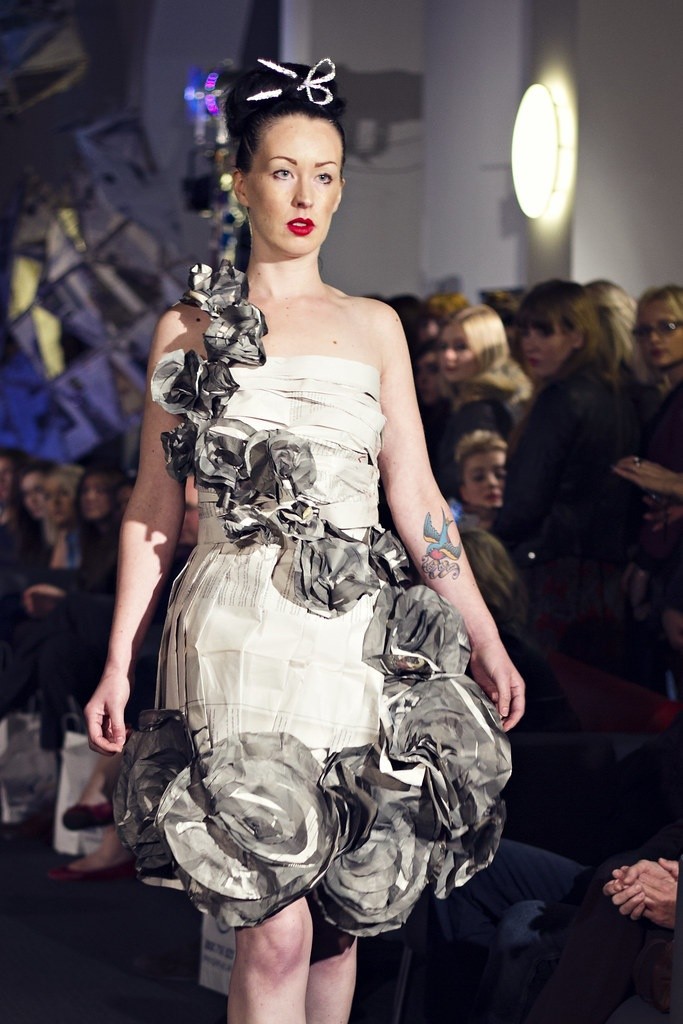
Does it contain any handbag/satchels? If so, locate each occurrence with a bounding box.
[1,691,56,826]
[196,911,236,996]
[53,730,123,855]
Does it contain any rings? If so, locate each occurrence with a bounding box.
[633,456,641,467]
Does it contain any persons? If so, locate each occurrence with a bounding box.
[83,56,528,1024]
[0,279,683,1024]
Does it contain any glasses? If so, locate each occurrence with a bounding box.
[632,320,683,339]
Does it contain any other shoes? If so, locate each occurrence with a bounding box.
[48,857,137,881]
[62,803,115,830]
